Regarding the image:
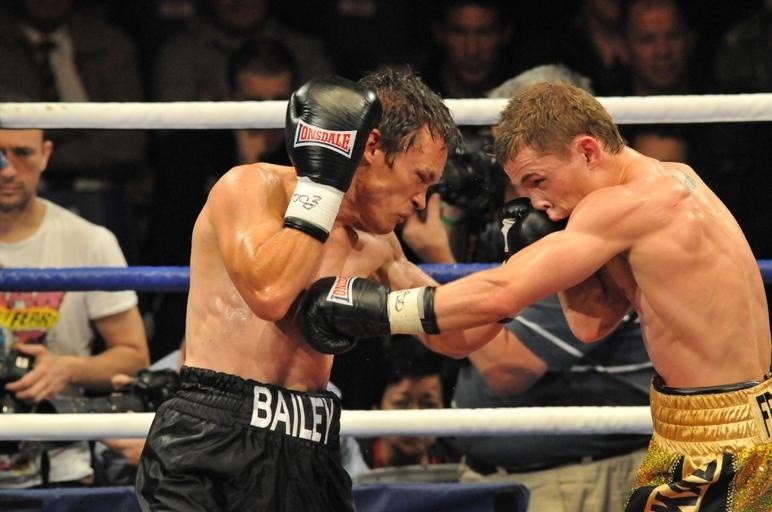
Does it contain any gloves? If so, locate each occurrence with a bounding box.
[497,197,569,257]
[299,275,440,355]
[283,73,382,243]
